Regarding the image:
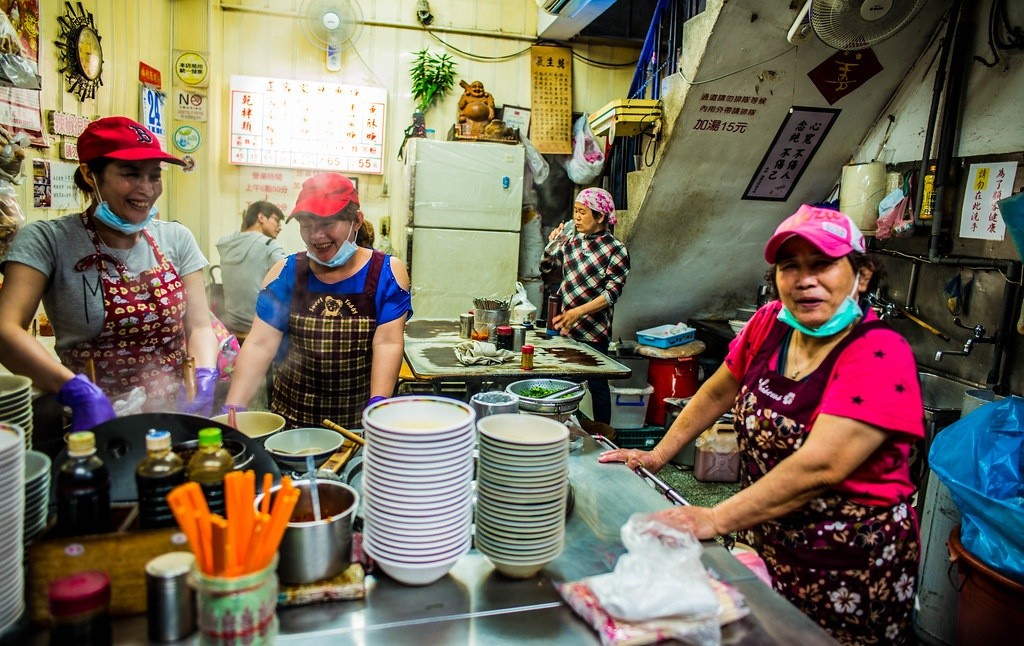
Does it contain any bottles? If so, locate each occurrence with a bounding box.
[135,429,234,532]
[57,431,110,540]
[460,314,474,339]
[497,326,534,370]
[919,166,936,219]
[48,550,198,646]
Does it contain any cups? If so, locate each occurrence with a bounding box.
[545,222,575,255]
[472,322,488,342]
[756,285,778,311]
[736,309,756,322]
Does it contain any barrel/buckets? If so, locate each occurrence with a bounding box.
[471,392,519,441]
[694,424,740,482]
[615,341,634,356]
[646,355,699,427]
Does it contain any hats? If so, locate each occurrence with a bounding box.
[765,204,867,266]
[77,117,188,167]
[284,173,359,224]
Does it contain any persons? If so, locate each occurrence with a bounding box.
[214,201,289,333]
[597,205,924,646]
[1,116,218,430]
[219,173,413,431]
[539,186,631,426]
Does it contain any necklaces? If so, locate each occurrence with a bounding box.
[791,330,829,378]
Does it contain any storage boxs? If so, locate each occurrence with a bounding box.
[613,354,650,384]
[578,382,654,428]
[661,398,696,468]
[635,323,697,348]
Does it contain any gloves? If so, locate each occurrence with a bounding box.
[219,404,247,416]
[176,367,220,419]
[369,396,384,405]
[56,372,117,430]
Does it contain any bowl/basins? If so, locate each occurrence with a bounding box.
[210,411,345,472]
[504,390,578,424]
[729,320,747,335]
[362,395,476,585]
[476,413,570,576]
[0,374,51,637]
[514,307,537,327]
[253,478,360,584]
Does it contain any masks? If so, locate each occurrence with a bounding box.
[775,272,866,338]
[306,222,361,268]
[91,170,158,235]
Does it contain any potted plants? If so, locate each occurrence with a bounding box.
[413,47,457,136]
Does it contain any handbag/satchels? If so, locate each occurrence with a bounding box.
[208,307,241,383]
[518,132,550,184]
[555,113,605,184]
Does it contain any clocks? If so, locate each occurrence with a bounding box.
[54,1,104,103]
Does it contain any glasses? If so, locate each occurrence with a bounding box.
[266,214,281,225]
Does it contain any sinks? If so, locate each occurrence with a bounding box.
[917,371,986,459]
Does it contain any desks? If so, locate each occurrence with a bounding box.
[403,318,633,407]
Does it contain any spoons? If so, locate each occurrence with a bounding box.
[273,447,323,455]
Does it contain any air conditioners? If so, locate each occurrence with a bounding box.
[536,0,579,18]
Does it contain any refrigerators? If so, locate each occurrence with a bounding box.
[396,137,525,319]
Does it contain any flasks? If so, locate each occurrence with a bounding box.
[546,294,562,335]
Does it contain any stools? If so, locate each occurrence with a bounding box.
[395,361,438,395]
[235,333,274,409]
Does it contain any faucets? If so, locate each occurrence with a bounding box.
[879,302,906,320]
[934,316,994,362]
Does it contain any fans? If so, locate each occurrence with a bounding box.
[297,0,365,73]
[786,0,927,52]
